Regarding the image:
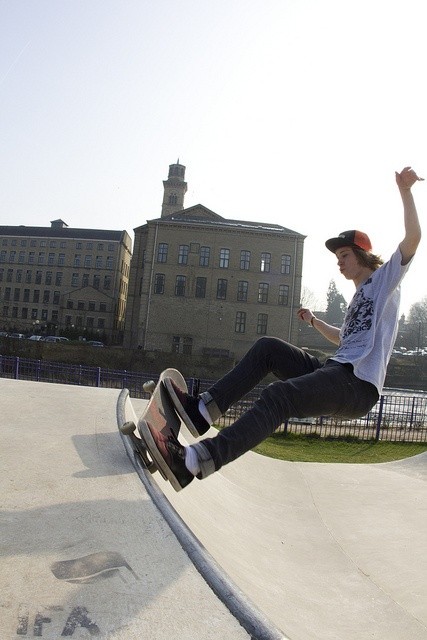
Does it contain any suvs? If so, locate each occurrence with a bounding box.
[44,337,69,344]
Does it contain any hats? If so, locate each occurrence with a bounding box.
[325,230,372,252]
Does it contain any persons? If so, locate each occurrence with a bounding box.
[138,164,426,493]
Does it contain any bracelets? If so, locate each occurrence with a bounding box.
[310,316,314,328]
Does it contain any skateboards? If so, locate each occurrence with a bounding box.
[120,368,188,481]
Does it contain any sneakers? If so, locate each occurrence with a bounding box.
[140,420,194,492]
[165,377,211,438]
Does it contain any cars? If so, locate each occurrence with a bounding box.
[88,341,104,347]
[27,336,44,341]
[9,333,25,338]
